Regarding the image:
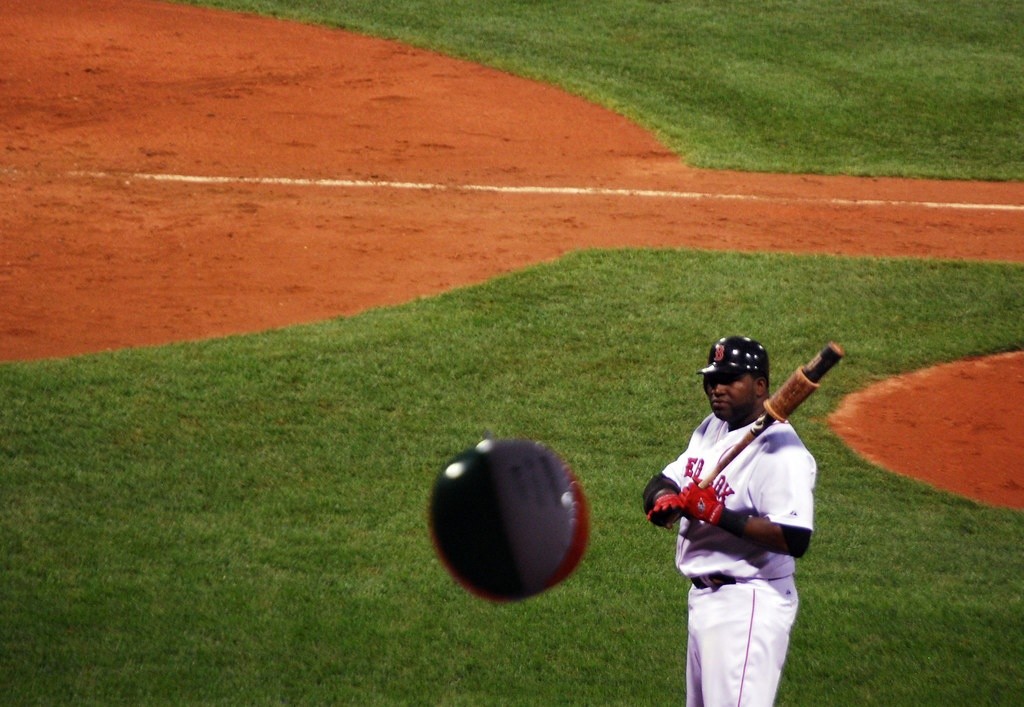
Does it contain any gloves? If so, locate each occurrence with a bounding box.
[648,488,685,529]
[676,477,724,527]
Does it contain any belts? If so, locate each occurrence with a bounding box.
[690,573,736,589]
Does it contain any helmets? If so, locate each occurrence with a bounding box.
[695,335,770,387]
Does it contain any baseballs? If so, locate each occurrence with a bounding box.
[429,436,591,604]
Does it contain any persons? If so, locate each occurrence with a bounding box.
[642,336,817,707]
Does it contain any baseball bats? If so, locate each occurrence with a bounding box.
[663,339,846,530]
